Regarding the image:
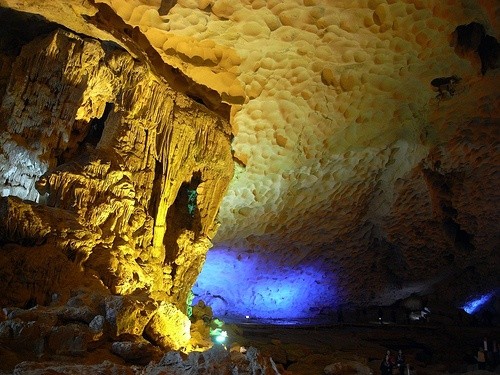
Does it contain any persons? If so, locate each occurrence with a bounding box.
[382,350,395,375]
[490,338,498,365]
[481,336,489,361]
[474,347,487,372]
[395,349,407,375]
[334,301,413,326]
